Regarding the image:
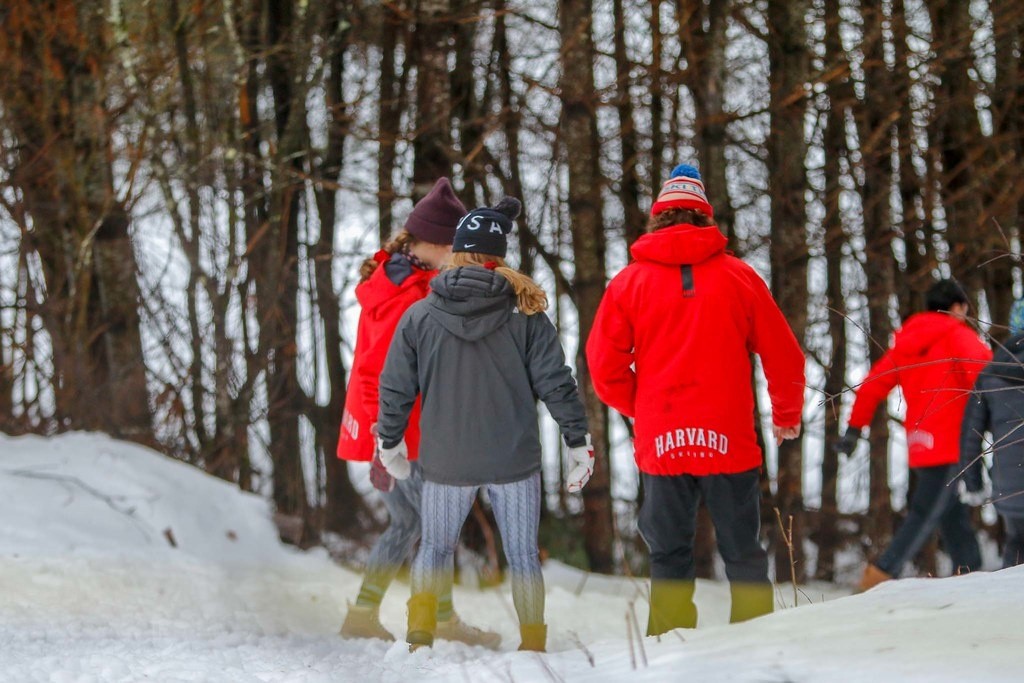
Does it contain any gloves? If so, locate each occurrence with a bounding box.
[376,436,413,479]
[369,423,397,491]
[965,478,982,490]
[838,427,862,454]
[562,433,597,493]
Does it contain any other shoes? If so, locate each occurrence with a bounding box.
[854,564,890,594]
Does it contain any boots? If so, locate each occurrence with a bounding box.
[518,624,547,651]
[406,594,438,646]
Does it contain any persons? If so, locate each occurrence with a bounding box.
[841,279,994,594]
[337,177,504,648]
[583,160,807,637]
[957,299,1024,569]
[377,196,596,652]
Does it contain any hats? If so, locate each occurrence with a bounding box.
[925,278,970,314]
[651,162,717,217]
[406,176,459,244]
[452,196,520,257]
[1010,299,1024,335]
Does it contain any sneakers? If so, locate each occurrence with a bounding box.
[437,613,502,649]
[340,610,398,642]
